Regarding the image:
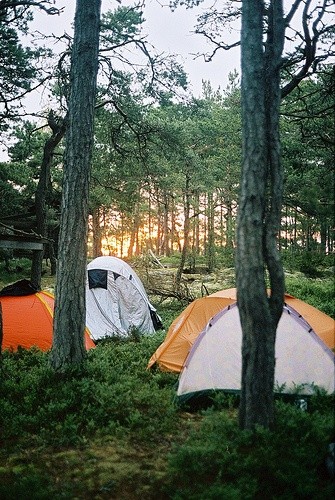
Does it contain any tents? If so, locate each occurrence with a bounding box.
[146,286,335,409]
[0,279,96,352]
[85,256,163,342]
